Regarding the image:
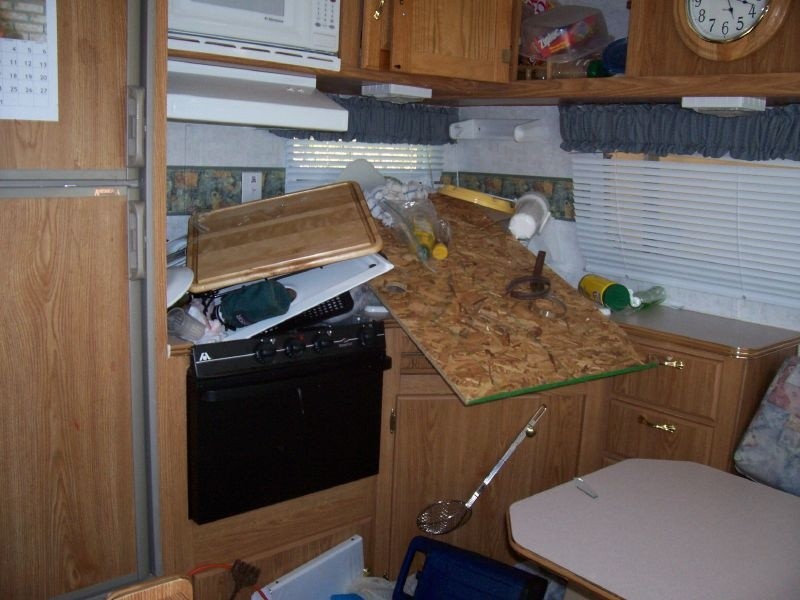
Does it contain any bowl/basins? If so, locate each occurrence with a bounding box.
[518,5,615,64]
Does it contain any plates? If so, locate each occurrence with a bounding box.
[437,184,515,214]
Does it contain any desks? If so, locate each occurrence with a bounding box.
[505,459,800,600]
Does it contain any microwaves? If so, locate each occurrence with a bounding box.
[165,0,342,72]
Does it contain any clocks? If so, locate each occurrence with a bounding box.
[674,0,790,62]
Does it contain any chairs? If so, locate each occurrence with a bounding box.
[392,535,549,600]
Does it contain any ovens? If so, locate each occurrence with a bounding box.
[186,321,392,527]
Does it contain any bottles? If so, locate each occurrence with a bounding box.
[509,191,551,240]
[630,286,666,307]
[577,274,629,311]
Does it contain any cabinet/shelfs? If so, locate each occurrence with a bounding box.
[0,0,800,600]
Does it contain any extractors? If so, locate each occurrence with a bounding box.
[165,55,349,132]
